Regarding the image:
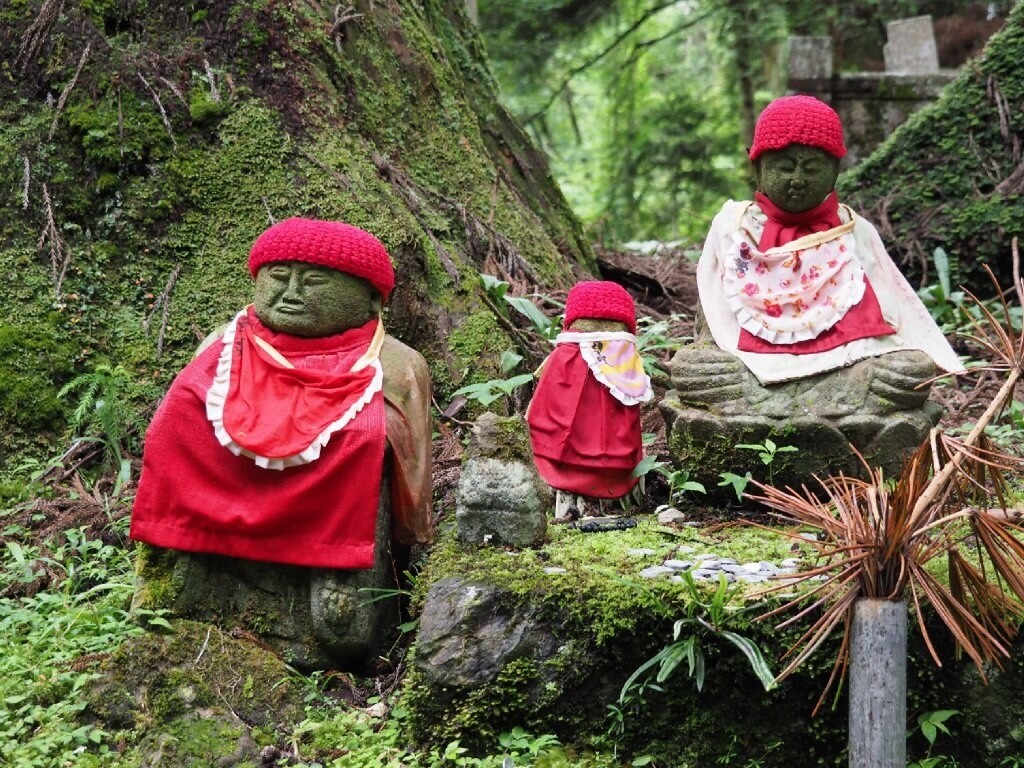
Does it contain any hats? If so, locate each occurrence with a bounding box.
[565,281,638,332]
[749,95,847,161]
[249,218,395,303]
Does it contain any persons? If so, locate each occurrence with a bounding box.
[127,219,430,665]
[526,282,656,525]
[669,96,966,420]
